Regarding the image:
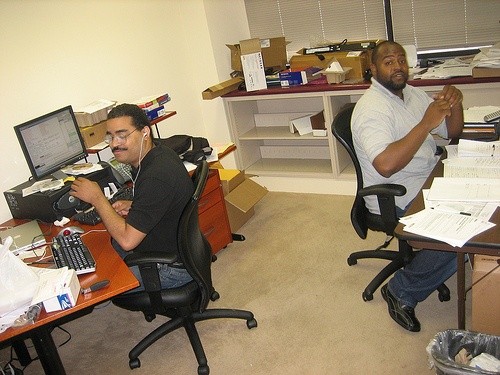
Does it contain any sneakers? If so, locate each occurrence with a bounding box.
[381,283,422,332]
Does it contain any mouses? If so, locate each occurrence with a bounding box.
[57,226,84,237]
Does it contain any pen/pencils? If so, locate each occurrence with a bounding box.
[491,145,495,156]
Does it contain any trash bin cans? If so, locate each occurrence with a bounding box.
[431,329,500,375]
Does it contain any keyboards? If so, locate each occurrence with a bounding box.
[74,186,135,225]
[51,234,97,276]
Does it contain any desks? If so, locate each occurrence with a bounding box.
[0,217,140,375]
[395,132,500,330]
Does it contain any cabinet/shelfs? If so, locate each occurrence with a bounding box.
[226,82,499,179]
[188,169,233,257]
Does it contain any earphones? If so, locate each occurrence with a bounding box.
[144,132,148,136]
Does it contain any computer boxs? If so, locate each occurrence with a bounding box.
[4,162,113,222]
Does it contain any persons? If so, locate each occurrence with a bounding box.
[350,41,464,332]
[68,104,194,294]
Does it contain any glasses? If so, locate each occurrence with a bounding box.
[104,124,145,145]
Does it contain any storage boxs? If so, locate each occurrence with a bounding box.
[472,65,500,78]
[201,34,383,100]
[219,169,268,234]
[289,114,314,134]
[80,120,107,149]
[310,109,328,136]
[146,106,166,122]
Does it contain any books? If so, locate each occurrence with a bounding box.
[138,94,171,122]
[414,64,472,79]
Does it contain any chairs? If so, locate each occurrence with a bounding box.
[332,103,452,302]
[112,160,257,375]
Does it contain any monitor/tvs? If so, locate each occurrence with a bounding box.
[13,105,88,179]
